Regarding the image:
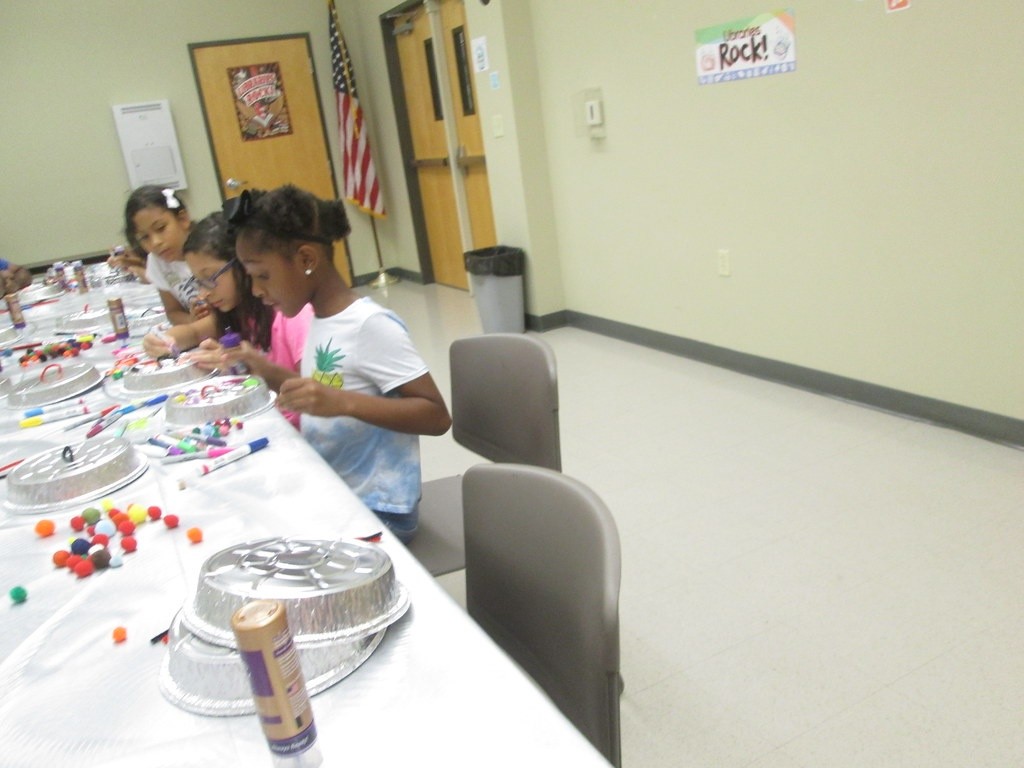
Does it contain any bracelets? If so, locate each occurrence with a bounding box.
[269,363,283,390]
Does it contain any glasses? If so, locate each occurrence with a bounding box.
[188,259,236,290]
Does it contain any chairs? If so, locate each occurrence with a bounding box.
[460,463,625,767]
[403,332,564,578]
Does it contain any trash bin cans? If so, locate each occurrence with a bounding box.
[464,245,527,334]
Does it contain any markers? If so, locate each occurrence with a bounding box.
[147,429,269,476]
[20,393,170,439]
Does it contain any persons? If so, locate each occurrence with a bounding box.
[0,257,35,301]
[107,227,151,284]
[211,184,452,547]
[140,211,319,431]
[122,185,216,328]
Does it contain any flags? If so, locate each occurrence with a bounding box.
[326,0,387,221]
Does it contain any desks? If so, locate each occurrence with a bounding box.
[0,259,614,767]
